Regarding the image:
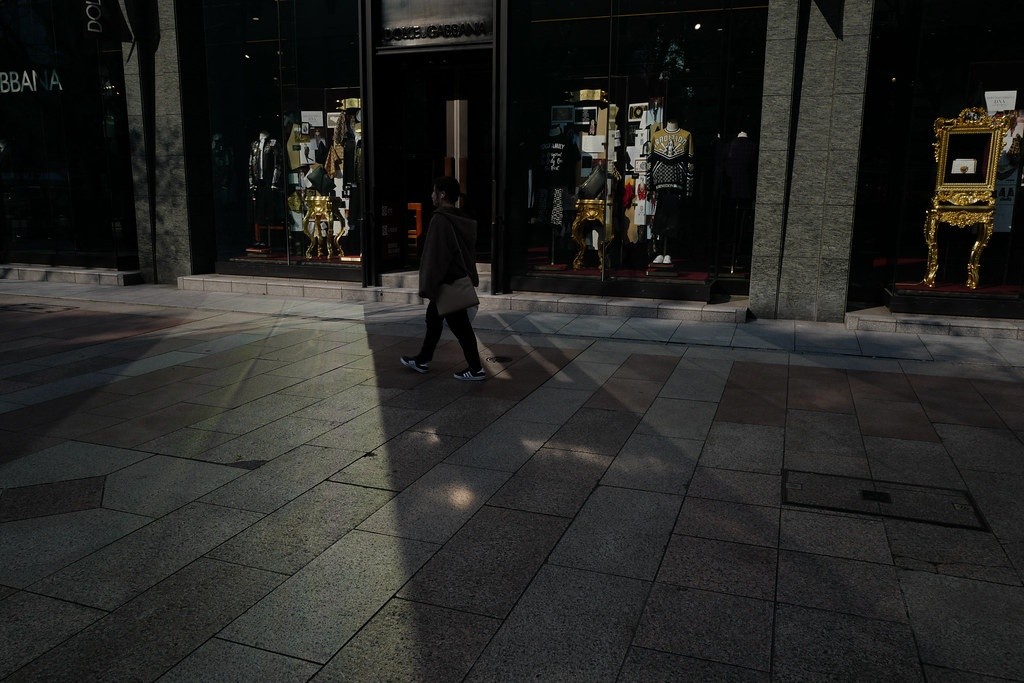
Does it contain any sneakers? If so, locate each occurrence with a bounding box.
[454,367,486,380]
[400,356,430,373]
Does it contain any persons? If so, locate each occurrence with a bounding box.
[399,174,487,381]
[646,118,693,265]
[247,129,285,259]
[527,124,581,228]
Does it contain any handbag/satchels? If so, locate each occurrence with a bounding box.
[307,165,336,196]
[436,276,480,315]
[579,165,606,199]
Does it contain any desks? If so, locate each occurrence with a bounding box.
[571,199,614,272]
[920,204,995,289]
[302,195,345,260]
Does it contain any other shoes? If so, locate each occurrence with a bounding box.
[653,255,663,263]
[663,255,672,263]
